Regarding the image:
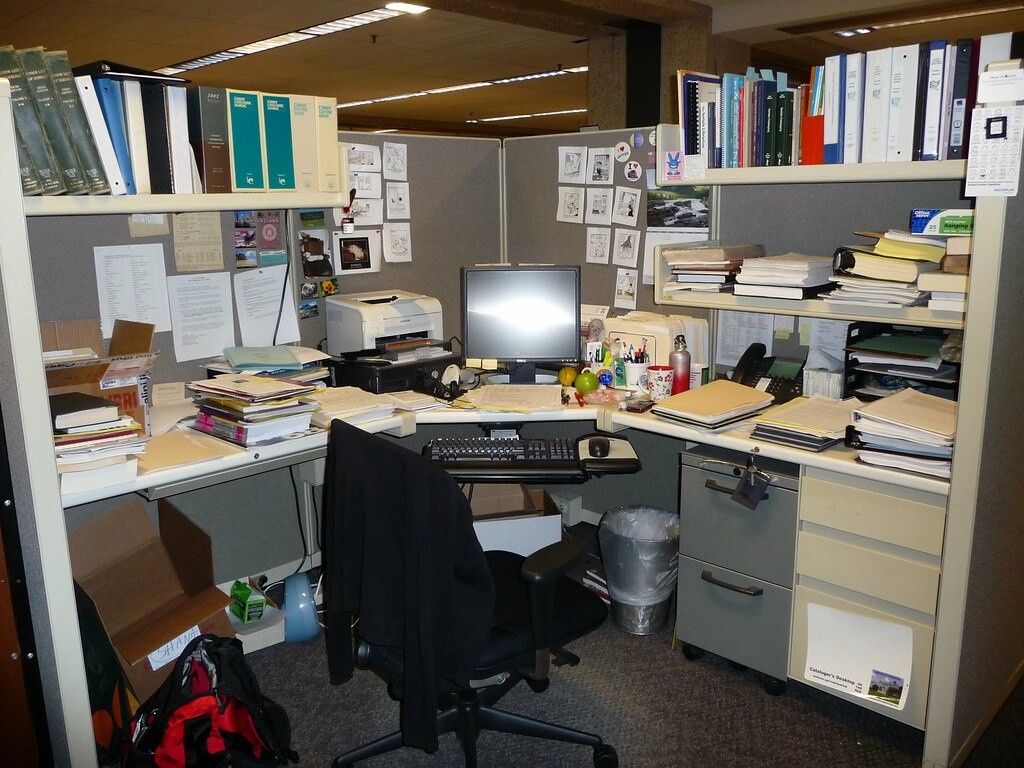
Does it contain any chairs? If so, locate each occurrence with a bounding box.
[320,416,620,768]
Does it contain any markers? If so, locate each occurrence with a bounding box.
[630,344,635,362]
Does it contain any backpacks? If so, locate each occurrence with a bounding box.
[117,633,297,768]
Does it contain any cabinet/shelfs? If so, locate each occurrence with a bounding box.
[604,60,1024,768]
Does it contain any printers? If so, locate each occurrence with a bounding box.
[325,289,452,357]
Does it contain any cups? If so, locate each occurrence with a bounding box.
[581,363,613,388]
[638,366,675,402]
[690,363,709,388]
[624,362,650,389]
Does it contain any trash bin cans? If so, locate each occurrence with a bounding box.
[603,506,680,635]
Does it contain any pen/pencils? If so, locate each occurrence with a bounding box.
[596,349,599,362]
[636,338,649,363]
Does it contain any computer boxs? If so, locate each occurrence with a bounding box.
[322,352,462,396]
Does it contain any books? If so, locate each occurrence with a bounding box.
[49,392,148,494]
[1,44,342,196]
[184,344,446,450]
[676,31,1023,168]
[649,230,973,482]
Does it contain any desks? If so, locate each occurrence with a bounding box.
[60,357,610,511]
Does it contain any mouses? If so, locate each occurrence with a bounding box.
[588,437,610,457]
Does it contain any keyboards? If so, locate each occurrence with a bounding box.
[422,437,580,468]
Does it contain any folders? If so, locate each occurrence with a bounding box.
[851,387,957,446]
[74,74,137,196]
[824,54,845,164]
[922,41,947,160]
[845,424,952,461]
[947,38,974,160]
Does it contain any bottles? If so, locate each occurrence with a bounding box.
[669,335,691,396]
[342,218,355,234]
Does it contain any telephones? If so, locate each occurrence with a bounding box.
[731,342,807,405]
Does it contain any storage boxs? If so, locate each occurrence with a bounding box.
[323,352,463,394]
[39,318,162,437]
[68,497,285,708]
[461,483,563,557]
[187,85,340,194]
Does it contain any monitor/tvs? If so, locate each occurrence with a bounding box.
[459,265,582,384]
